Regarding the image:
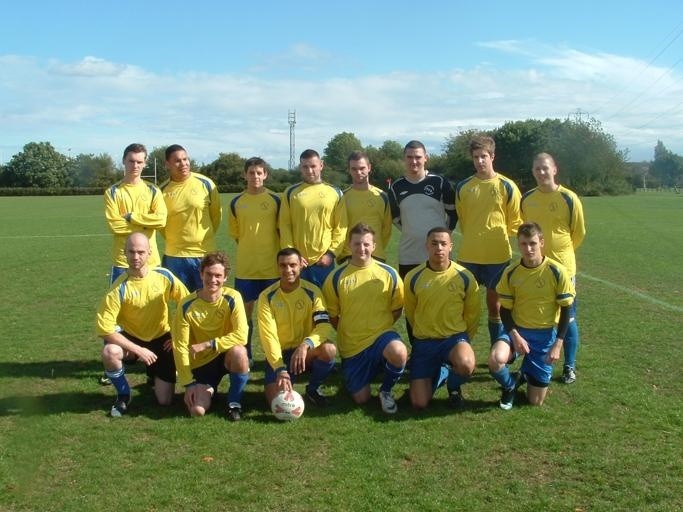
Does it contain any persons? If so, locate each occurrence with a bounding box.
[227,156,282,368]
[454,136,523,345]
[278,149,349,288]
[172,253,248,422]
[403,227,475,409]
[520,151,585,386]
[343,151,393,258]
[388,139,458,350]
[158,146,222,293]
[321,223,406,415]
[488,222,577,412]
[97,232,192,416]
[255,246,336,408]
[99,143,167,390]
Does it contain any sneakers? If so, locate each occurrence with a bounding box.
[111,391,131,418]
[448,386,465,410]
[499,380,516,410]
[305,385,332,408]
[223,400,242,421]
[562,365,576,384]
[380,390,397,414]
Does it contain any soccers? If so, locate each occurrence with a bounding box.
[271,390,304,420]
